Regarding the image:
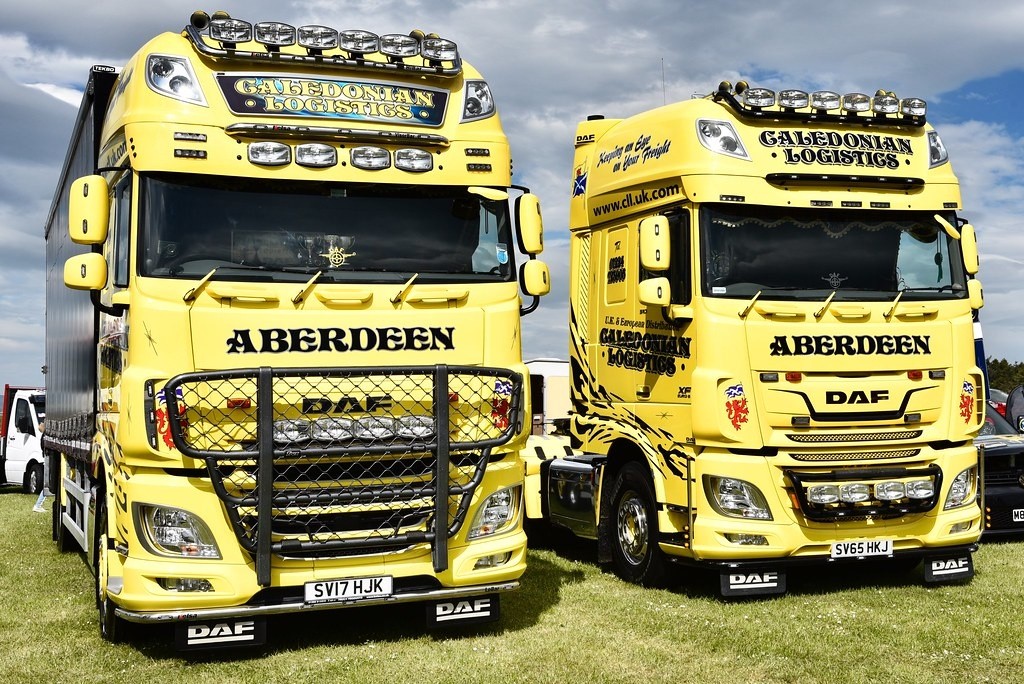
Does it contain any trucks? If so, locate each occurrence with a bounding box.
[0,384,48,494]
[40,9,549,661]
[525,82,985,601]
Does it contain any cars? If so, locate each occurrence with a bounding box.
[974,384,1024,540]
